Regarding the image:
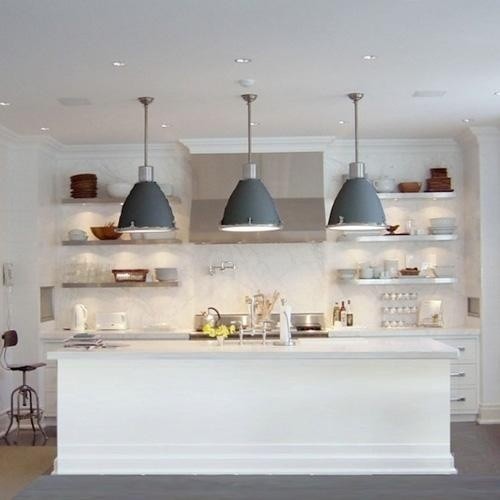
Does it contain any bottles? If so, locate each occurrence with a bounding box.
[339,301,345,322]
[333,303,339,324]
[345,300,354,327]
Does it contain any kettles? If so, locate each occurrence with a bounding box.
[71,303,87,331]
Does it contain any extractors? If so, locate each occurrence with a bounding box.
[189,152,326,245]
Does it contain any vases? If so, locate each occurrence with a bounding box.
[217,337,225,347]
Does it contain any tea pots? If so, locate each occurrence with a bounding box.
[372,177,395,192]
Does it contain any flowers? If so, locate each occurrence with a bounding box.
[203,324,235,337]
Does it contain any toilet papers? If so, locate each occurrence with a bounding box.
[279,305,291,341]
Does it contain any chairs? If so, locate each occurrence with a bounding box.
[0,330,49,444]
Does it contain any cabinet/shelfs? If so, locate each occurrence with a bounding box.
[58,197,182,287]
[327,325,480,423]
[336,192,458,285]
[39,332,190,434]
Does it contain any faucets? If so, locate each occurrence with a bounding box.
[250,294,264,333]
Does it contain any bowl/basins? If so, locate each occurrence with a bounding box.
[399,182,421,192]
[399,268,421,275]
[68,234,88,240]
[336,269,356,280]
[430,267,457,277]
[90,226,122,240]
[67,229,87,234]
[429,217,457,225]
[106,182,132,197]
[427,224,457,234]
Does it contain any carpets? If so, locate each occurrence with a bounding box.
[7,421,499,499]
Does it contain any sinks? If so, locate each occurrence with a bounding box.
[258,324,327,339]
[193,328,254,340]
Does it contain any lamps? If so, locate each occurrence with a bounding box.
[114,96,177,233]
[326,93,387,230]
[221,94,283,232]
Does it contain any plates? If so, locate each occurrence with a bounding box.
[69,174,98,198]
[401,276,420,278]
[155,268,177,281]
[359,267,373,279]
[424,167,454,192]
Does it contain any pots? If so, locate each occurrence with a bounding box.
[290,313,324,329]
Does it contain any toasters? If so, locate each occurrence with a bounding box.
[95,312,129,330]
